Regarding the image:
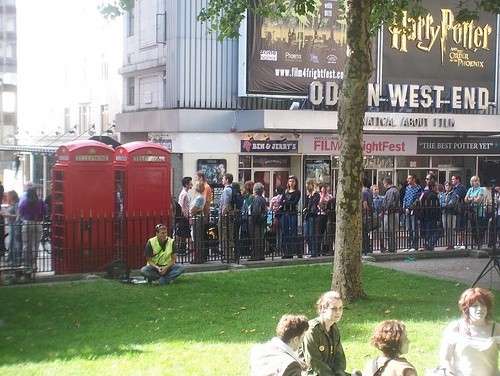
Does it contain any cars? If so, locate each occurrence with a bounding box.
[208,206,221,225]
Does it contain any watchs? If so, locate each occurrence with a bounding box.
[155,266,158,269]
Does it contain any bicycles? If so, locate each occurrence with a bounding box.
[3,214,51,254]
[171,226,195,256]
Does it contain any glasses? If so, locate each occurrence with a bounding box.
[426,178,431,180]
[289,175,296,178]
[156,224,163,232]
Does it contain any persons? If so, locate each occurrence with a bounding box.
[425,286,500,376]
[140,223,184,286]
[297,291,351,376]
[248,314,309,376]
[0,171,500,273]
[353,320,417,376]
